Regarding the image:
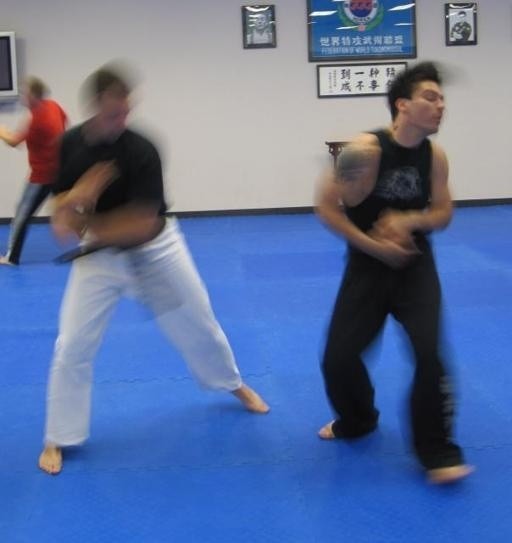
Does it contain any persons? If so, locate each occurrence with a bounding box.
[1,77,67,265]
[314,62,473,485]
[248,13,272,43]
[39,64,272,474]
[450,10,471,40]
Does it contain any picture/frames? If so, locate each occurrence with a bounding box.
[316,61,409,99]
[445,2,478,46]
[240,2,278,50]
[305,0,418,64]
